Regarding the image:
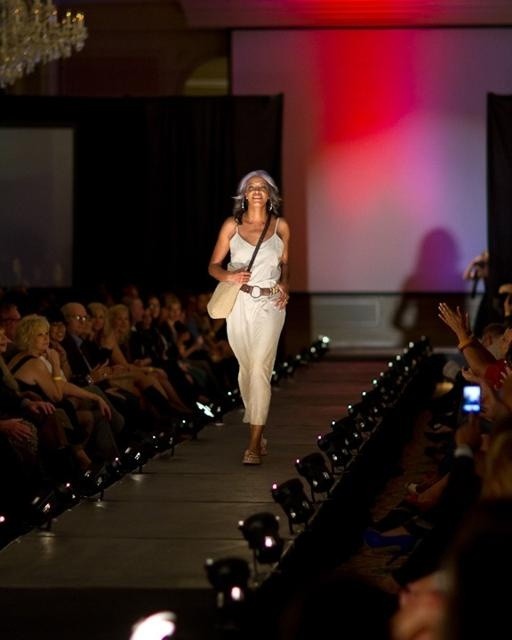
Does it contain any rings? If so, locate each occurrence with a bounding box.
[284,298,288,304]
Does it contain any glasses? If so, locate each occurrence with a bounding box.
[66,315,88,321]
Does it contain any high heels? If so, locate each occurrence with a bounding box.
[366,523,415,566]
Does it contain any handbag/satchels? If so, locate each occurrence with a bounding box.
[207,262,243,320]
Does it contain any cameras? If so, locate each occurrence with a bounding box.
[474,261,485,268]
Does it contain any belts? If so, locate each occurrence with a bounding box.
[240,284,280,298]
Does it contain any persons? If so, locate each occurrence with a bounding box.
[207,169,291,465]
[0,285,237,542]
[370,255,512,640]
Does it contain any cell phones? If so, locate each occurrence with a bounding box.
[460,382,482,414]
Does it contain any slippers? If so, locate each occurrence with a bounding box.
[260,433,268,456]
[242,449,261,464]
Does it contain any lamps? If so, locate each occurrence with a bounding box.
[294,453,336,494]
[361,387,387,415]
[227,386,242,406]
[29,496,58,531]
[331,416,364,450]
[347,399,377,432]
[316,432,353,467]
[114,446,143,476]
[270,477,315,530]
[374,341,422,406]
[195,394,224,427]
[204,554,253,601]
[150,428,176,456]
[76,468,108,501]
[0,1,92,87]
[271,336,331,384]
[177,413,198,441]
[237,511,286,567]
